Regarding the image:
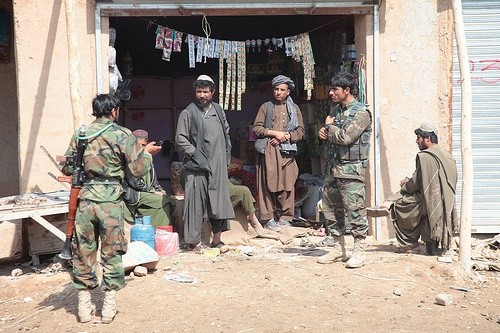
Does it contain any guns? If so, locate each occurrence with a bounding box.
[57,124,89,261]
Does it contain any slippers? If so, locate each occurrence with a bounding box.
[366,205,391,217]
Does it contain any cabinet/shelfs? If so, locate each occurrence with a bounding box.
[294,25,356,176]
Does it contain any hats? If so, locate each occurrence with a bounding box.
[419,119,439,136]
[272,75,295,91]
[197,75,214,84]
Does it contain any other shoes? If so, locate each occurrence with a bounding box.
[187,242,204,250]
[264,219,278,229]
[278,220,293,227]
[210,242,224,248]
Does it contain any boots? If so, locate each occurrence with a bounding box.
[317,234,346,265]
[100,289,118,324]
[344,237,367,268]
[77,288,96,323]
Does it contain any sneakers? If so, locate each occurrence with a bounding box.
[316,236,335,247]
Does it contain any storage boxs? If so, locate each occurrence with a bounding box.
[248,50,284,74]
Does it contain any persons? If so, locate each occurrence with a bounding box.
[391,123,458,254]
[61,93,164,324]
[121,74,373,269]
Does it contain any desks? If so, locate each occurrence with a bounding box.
[0,190,70,266]
